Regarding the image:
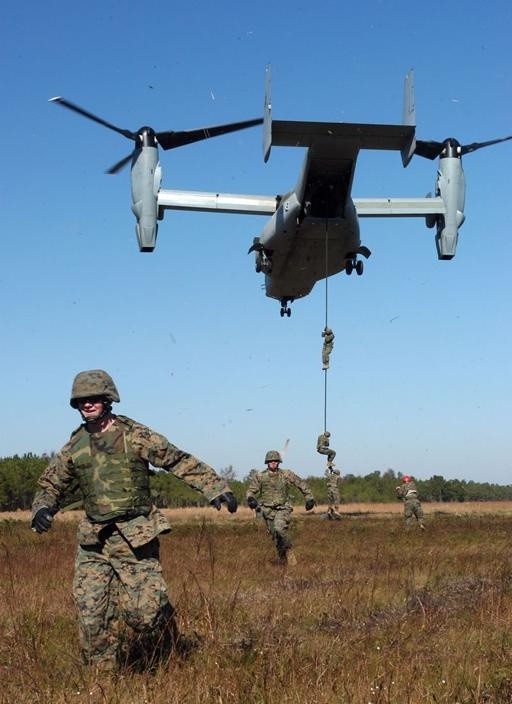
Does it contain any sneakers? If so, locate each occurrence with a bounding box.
[286,548,297,567]
[420,524,425,529]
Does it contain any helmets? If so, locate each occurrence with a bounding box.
[402,476,410,482]
[70,370,119,409]
[333,469,340,475]
[324,432,330,437]
[265,451,282,462]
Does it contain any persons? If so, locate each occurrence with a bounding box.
[321,326,334,370]
[316,431,337,465]
[396,474,426,531]
[246,450,318,566]
[323,466,343,520]
[29,368,239,670]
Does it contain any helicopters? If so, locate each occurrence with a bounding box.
[48,60,512,318]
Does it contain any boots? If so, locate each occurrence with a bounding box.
[327,461,335,466]
[323,362,328,370]
[328,508,341,517]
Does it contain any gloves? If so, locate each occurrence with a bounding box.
[214,493,237,513]
[305,499,314,510]
[32,507,59,534]
[248,497,257,509]
